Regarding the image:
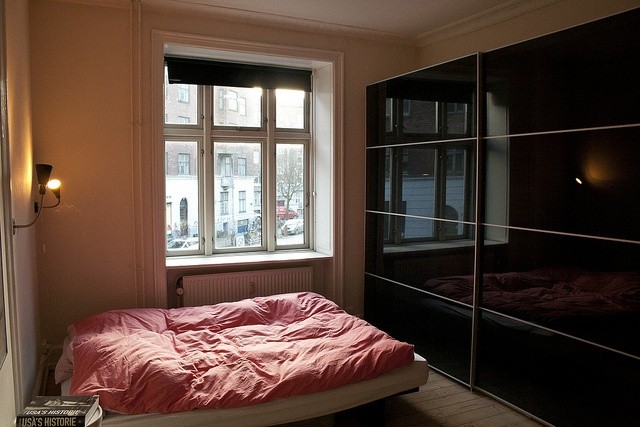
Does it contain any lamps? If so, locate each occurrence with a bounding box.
[12,163,54,233]
[34,178,62,214]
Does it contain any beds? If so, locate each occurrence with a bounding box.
[35,291,429,427]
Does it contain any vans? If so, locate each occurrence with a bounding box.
[275,206,298,220]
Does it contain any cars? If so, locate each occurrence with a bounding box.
[281,219,303,236]
[167,239,193,249]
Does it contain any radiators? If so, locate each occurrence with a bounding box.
[182,265,314,306]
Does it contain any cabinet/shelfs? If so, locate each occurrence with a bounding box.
[362,7,640,426]
